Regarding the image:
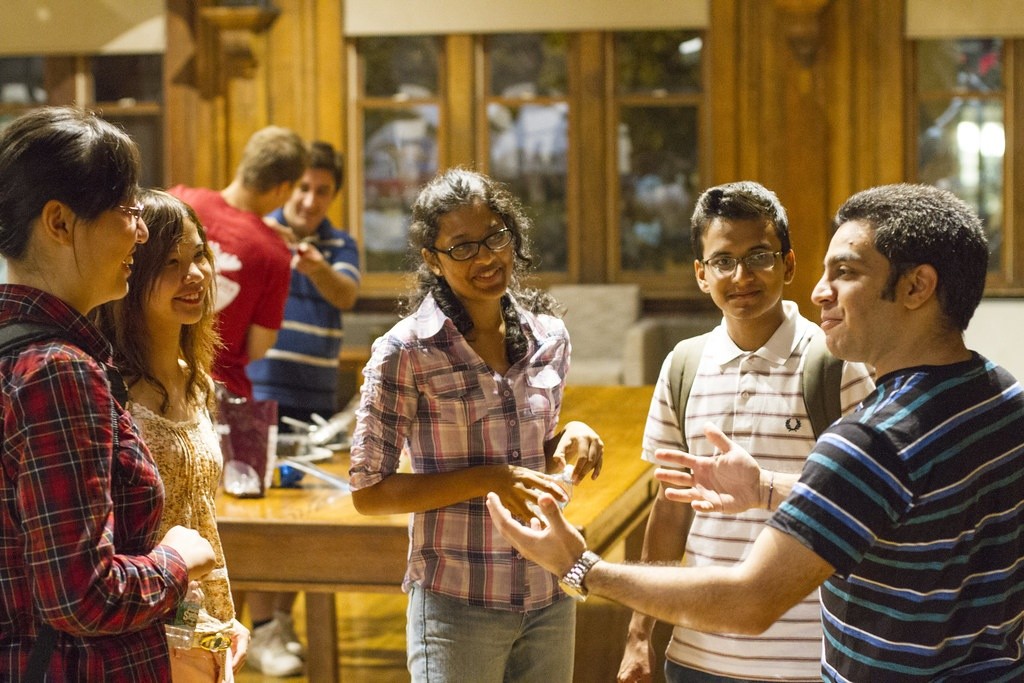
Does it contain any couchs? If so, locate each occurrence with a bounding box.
[547,283,663,384]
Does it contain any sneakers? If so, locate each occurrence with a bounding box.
[243,618,302,678]
[273,611,304,657]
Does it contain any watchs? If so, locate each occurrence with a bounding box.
[557,548,602,603]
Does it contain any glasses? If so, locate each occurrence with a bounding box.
[107,204,142,222]
[702,249,783,273]
[426,219,513,261]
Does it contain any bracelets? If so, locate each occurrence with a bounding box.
[764,471,776,512]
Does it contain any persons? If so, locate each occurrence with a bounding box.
[163,128,310,404]
[486,183,1024,682]
[90,183,251,682]
[1,106,218,681]
[346,168,605,682]
[243,136,361,678]
[612,178,878,683]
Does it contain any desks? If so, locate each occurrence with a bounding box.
[216,385,659,683]
